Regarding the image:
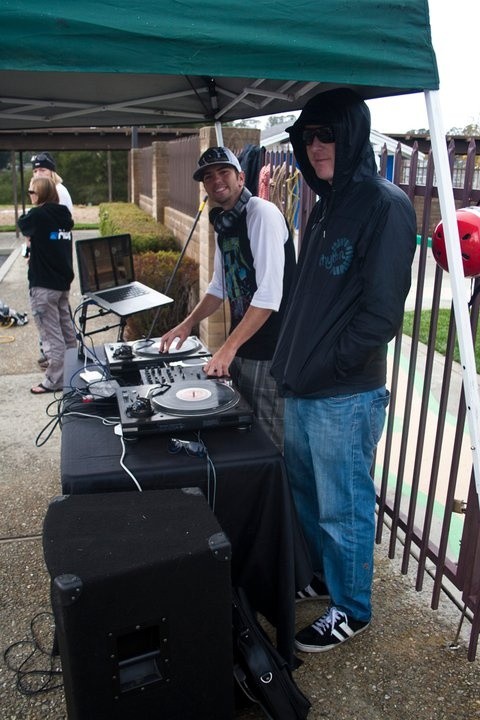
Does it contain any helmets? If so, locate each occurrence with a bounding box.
[431,205,480,278]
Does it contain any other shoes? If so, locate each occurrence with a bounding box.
[38,357,49,368]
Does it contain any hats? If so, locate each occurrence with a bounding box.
[32,152,56,172]
[192,147,242,180]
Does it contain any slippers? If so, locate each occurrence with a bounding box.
[30,383,63,393]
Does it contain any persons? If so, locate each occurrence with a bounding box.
[26,151,74,370]
[16,177,78,396]
[268,85,415,653]
[160,147,297,458]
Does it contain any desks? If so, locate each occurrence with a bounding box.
[77,299,131,367]
[62,345,297,664]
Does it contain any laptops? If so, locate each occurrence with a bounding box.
[76,234,174,317]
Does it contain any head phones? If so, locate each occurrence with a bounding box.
[210,186,251,236]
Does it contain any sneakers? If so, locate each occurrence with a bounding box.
[293,577,331,603]
[294,605,370,652]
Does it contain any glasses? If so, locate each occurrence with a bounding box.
[27,190,35,194]
[298,126,334,145]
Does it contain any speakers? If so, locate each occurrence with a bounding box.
[40,485,238,719]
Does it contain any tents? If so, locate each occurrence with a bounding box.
[1,0,480,506]
